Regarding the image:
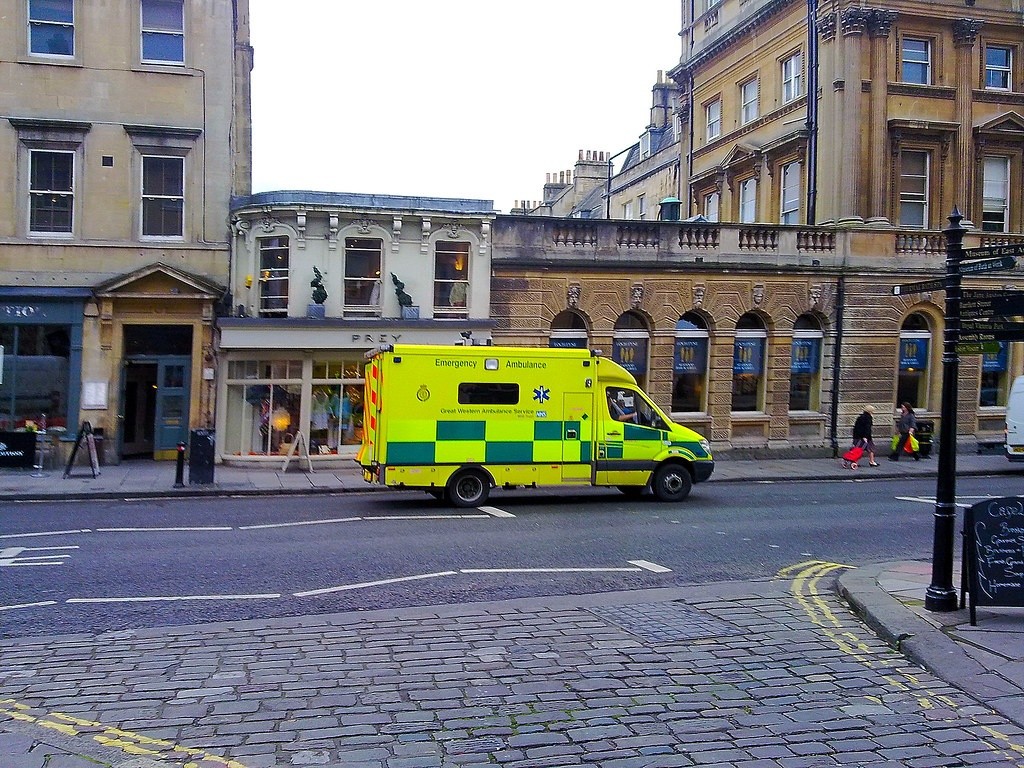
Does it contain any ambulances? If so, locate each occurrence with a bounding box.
[357,336,711,507]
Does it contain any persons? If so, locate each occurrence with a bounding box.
[886,402,919,461]
[333,388,351,441]
[607,397,637,421]
[310,388,336,445]
[842,405,880,469]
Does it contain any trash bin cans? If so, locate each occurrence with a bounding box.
[915,420,934,459]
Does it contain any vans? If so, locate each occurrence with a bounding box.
[1001,373,1024,462]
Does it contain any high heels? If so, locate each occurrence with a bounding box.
[869,462,880,467]
[850,462,861,467]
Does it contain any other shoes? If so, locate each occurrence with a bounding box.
[888,457,898,461]
[913,457,919,461]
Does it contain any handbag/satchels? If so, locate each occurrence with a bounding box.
[910,434,919,452]
[318,444,331,454]
[903,435,913,454]
[890,433,900,452]
[278,433,298,455]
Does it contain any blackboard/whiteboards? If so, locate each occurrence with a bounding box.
[963,497,1024,607]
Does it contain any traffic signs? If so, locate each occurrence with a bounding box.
[891,241,1022,359]
